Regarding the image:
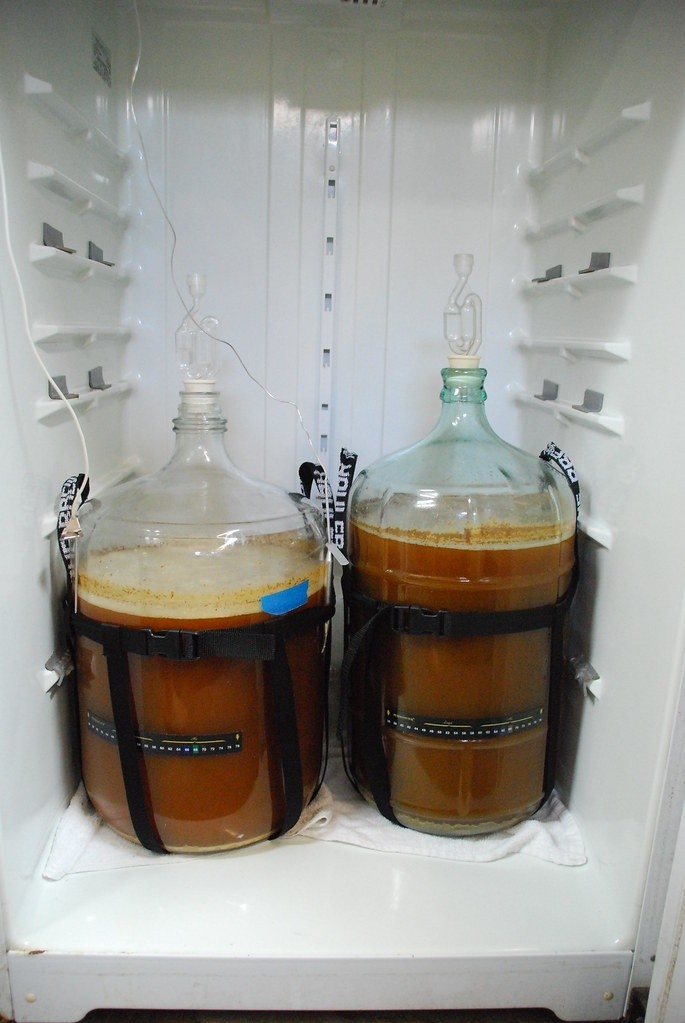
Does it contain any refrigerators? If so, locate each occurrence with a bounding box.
[2,3,683,1022]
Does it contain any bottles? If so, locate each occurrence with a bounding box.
[71,381,327,855]
[343,353,576,841]
